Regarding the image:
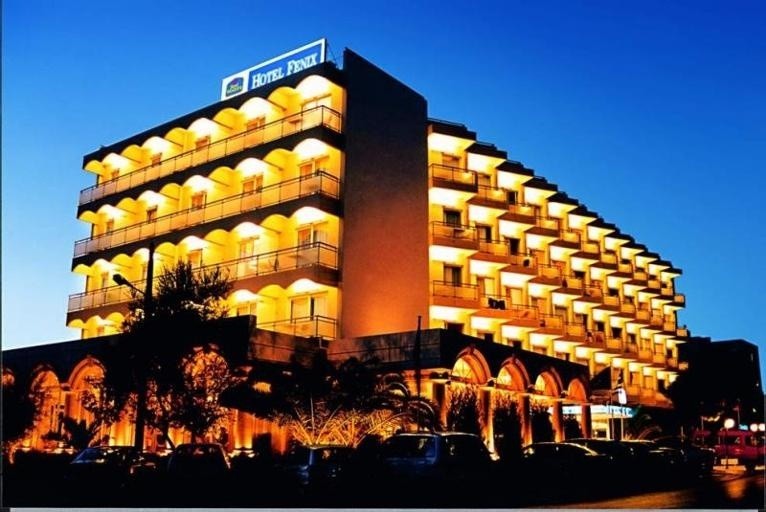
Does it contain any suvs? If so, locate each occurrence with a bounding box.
[715,429,766,471]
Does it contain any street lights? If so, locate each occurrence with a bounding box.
[609,386,622,438]
[111,271,152,451]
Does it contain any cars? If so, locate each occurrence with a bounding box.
[271,435,711,503]
[167,442,231,474]
[68,446,156,476]
